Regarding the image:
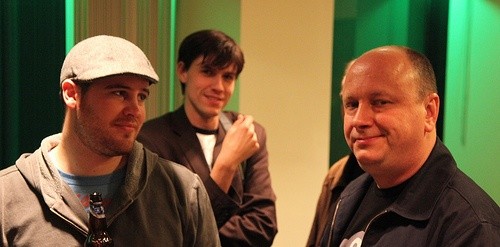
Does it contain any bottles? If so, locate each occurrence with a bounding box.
[84,192,114,247]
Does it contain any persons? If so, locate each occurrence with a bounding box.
[319,43,500,247]
[305,58,367,247]
[134,28,279,247]
[0,34,223,247]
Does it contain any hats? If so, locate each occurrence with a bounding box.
[60,34,159,86]
